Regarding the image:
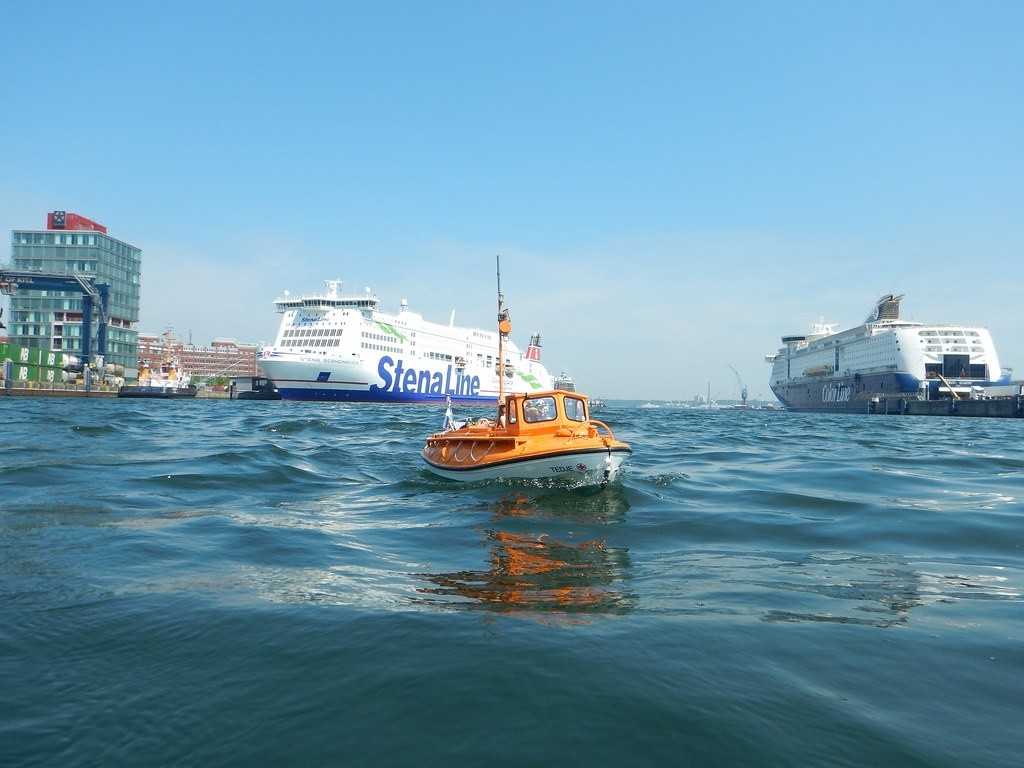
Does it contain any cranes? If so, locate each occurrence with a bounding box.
[0,264,110,384]
[729,365,748,405]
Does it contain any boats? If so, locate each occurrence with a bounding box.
[234,374,282,401]
[803,364,834,376]
[116,324,199,400]
[637,381,786,412]
[417,251,635,490]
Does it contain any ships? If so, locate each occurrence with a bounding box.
[763,291,1024,418]
[253,277,578,405]
